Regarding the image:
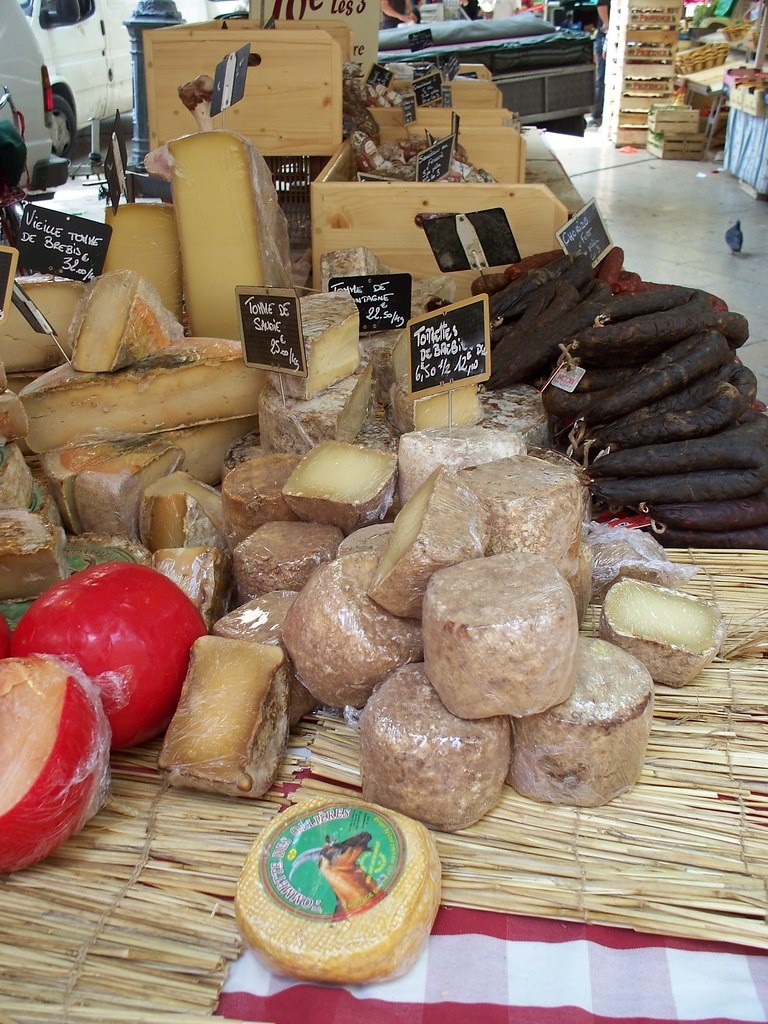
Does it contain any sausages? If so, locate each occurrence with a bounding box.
[472,247,768,550]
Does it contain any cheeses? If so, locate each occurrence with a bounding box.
[0,136,721,986]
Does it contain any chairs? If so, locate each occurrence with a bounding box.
[122,171,171,203]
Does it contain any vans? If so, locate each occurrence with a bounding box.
[18,0,249,158]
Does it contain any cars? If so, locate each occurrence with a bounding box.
[0,1,72,202]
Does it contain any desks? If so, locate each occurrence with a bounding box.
[521,127,583,214]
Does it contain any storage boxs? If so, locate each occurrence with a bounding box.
[371,61,513,123]
[297,126,569,303]
[747,30,760,48]
[721,66,768,116]
[610,0,683,26]
[260,154,328,194]
[604,76,675,94]
[606,57,676,79]
[646,105,701,134]
[605,109,648,127]
[277,195,311,250]
[141,17,351,156]
[605,42,677,60]
[608,25,680,45]
[601,124,647,150]
[645,130,706,161]
[604,92,675,112]
